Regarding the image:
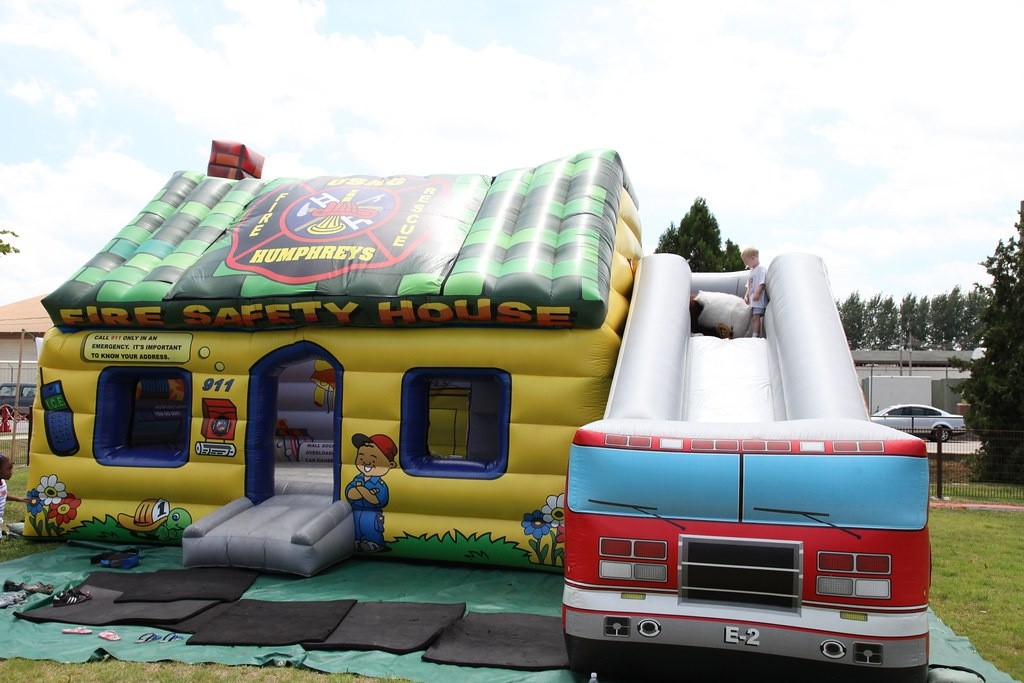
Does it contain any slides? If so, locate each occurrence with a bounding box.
[601,254,874,421]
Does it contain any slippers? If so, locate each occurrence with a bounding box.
[158,632,184,643]
[61,625,93,634]
[98,629,121,641]
[134,632,161,643]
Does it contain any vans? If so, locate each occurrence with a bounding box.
[0,382,37,414]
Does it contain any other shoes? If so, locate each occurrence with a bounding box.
[0,592,28,608]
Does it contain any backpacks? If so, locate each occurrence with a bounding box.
[100,548,141,567]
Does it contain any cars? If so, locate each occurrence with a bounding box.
[870,403,966,442]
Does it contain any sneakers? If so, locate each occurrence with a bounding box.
[52,591,85,606]
[67,589,92,600]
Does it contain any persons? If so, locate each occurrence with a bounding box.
[0,454,13,541]
[742,250,769,337]
[690,283,733,339]
